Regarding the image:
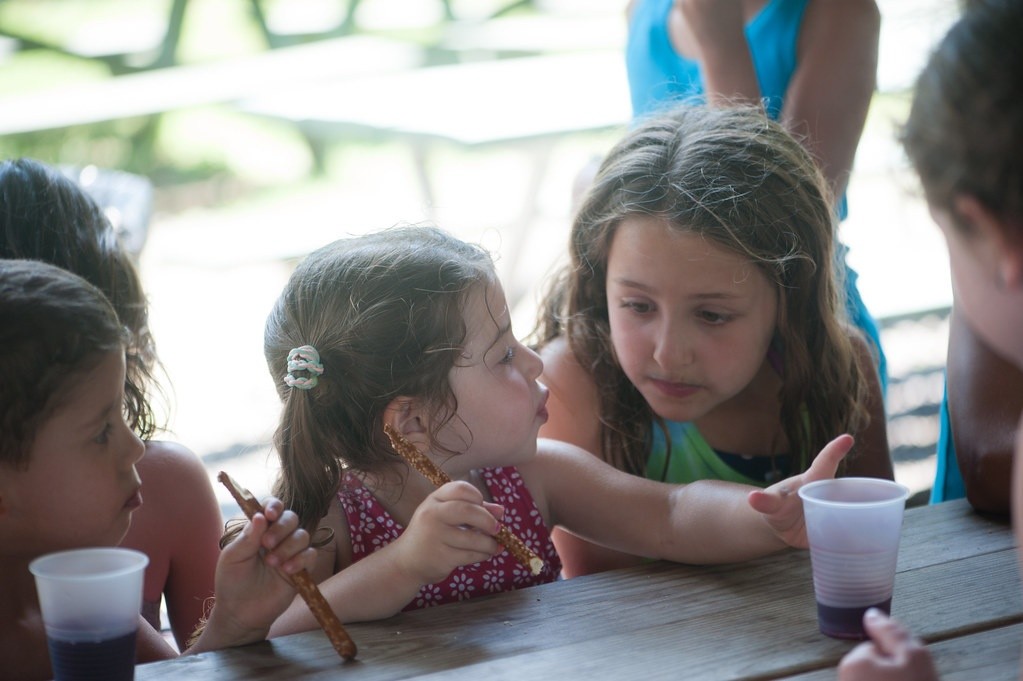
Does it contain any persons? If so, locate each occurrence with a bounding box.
[524,106,893,581]
[837,9,1023,681]
[624,0,889,403]
[0,260,315,681]
[218,225,855,641]
[0,158,225,655]
[927,0,1023,524]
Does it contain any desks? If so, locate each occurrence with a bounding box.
[132,497,1023,681]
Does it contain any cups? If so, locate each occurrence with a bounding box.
[798,477,909,640]
[30,548,149,681]
